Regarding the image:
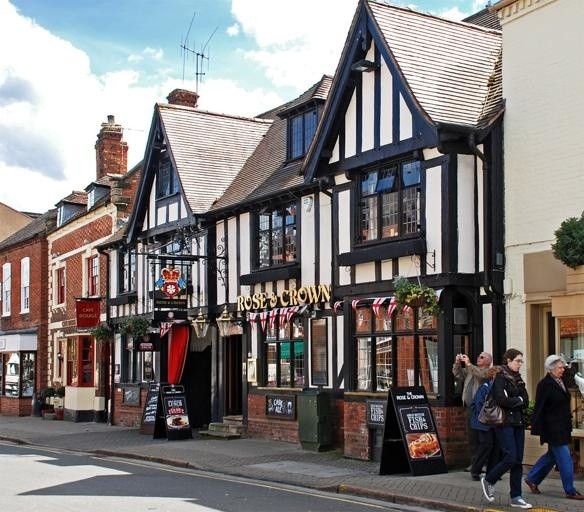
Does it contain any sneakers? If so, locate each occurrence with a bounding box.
[510,495,532,509]
[480,476,495,502]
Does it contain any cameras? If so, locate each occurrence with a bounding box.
[458,353,463,359]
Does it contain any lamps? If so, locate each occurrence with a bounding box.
[188,258,237,339]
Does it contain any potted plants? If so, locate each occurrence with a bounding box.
[393,276,447,322]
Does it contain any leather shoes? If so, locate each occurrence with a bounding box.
[524,478,541,494]
[471,471,481,481]
[566,491,584,500]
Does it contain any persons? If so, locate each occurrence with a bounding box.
[452,351,493,473]
[470,366,503,481]
[524,354,584,500]
[480,348,533,509]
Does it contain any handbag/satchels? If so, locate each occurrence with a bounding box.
[478,372,507,428]
